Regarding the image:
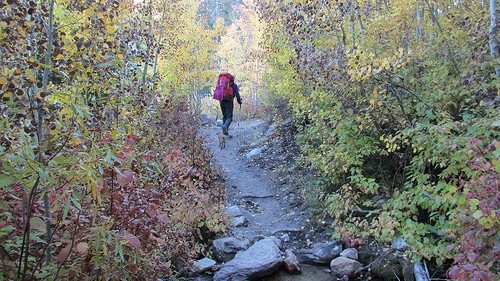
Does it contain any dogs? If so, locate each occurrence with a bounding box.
[216,132,226,149]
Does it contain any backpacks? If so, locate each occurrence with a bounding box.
[213,71,234,99]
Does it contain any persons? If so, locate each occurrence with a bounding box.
[219,75,243,135]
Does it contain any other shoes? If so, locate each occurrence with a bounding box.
[223,124,227,134]
